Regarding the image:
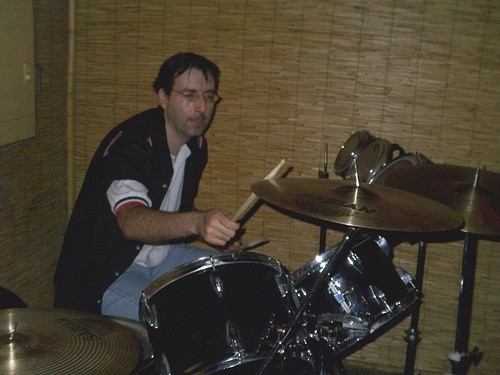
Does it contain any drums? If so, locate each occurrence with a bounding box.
[334,130,378,179]
[140,253,316,375]
[345,139,405,182]
[104,314,155,375]
[369,152,433,185]
[291,234,418,358]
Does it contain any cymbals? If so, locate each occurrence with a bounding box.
[385,164,500,234]
[0,309,141,375]
[250,177,464,232]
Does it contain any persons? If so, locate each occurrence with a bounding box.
[53,51,243,322]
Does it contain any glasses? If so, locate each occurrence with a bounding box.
[171,88,222,104]
